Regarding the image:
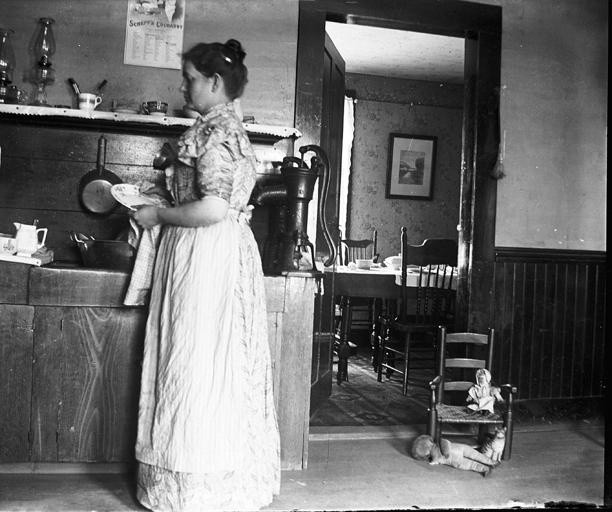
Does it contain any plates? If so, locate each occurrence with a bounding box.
[113,108,140,114]
[412,268,437,275]
[111,183,171,211]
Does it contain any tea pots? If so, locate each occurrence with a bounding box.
[12,222,48,258]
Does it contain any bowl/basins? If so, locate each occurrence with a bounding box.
[355,259,373,268]
[383,256,402,270]
[69,230,137,274]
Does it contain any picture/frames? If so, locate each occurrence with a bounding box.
[384,132,438,200]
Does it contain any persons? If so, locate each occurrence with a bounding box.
[465,367,505,417]
[408,429,498,479]
[123,36,284,507]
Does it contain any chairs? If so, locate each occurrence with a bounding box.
[378,227,456,396]
[332,226,384,364]
[427,325,518,460]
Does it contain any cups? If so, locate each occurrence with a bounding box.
[142,100,168,117]
[79,92,102,110]
[0,233,18,256]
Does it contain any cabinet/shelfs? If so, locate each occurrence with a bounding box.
[32,261,316,474]
[1,261,34,474]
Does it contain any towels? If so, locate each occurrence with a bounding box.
[122,179,162,307]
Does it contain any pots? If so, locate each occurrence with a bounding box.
[77,137,124,219]
